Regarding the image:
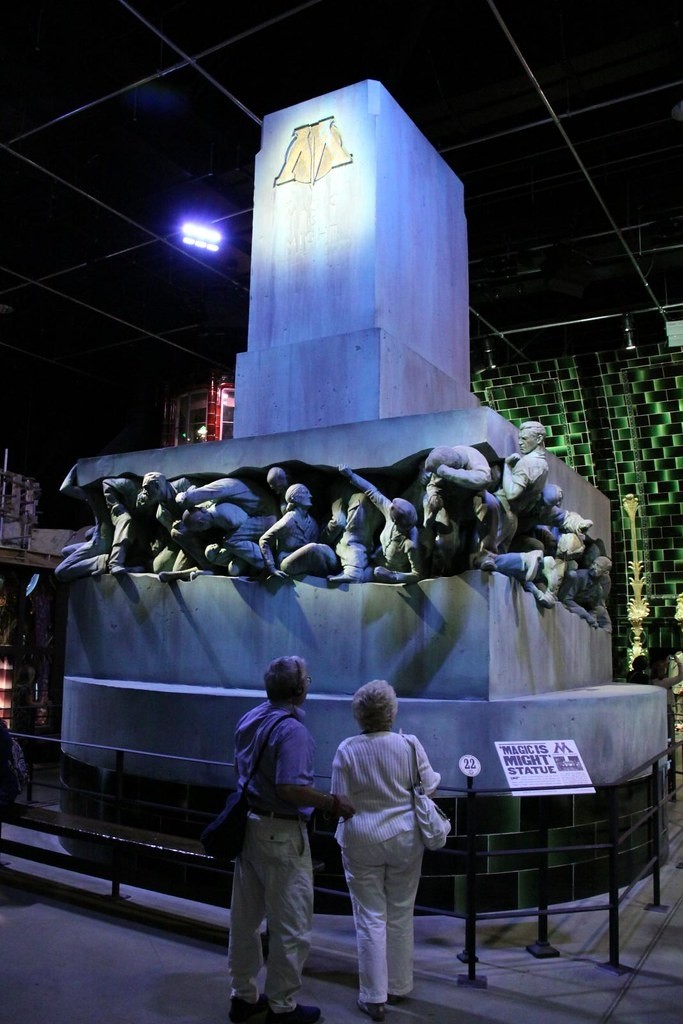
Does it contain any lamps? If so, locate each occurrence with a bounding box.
[483,336,492,352]
[621,315,637,351]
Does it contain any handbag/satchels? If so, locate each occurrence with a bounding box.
[402,733,451,851]
[200,792,248,864]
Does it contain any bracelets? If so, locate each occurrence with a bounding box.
[317,792,338,812]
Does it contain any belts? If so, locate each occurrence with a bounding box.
[249,805,309,823]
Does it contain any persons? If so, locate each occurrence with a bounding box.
[331,680,440,1020]
[227,654,357,1024]
[627,652,683,766]
[55,421,610,631]
[0,665,48,862]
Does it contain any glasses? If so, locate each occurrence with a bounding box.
[299,676,312,687]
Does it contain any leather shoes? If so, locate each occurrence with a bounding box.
[357,998,385,1022]
[385,994,398,1005]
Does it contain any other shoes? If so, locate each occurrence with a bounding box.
[0,860,12,868]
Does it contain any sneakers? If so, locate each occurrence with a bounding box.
[228,993,269,1023]
[264,1003,321,1024]
